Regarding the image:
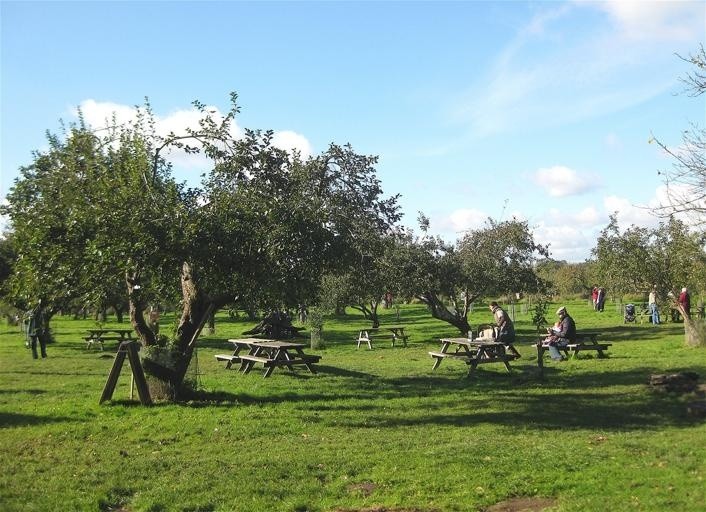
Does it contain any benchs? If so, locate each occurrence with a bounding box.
[214,338,322,378]
[81,330,140,351]
[638,304,706,323]
[428,337,518,376]
[356,327,410,349]
[532,332,612,361]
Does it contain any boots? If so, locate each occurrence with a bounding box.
[506,344,521,360]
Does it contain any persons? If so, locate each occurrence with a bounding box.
[678,287,691,320]
[489,301,521,360]
[667,285,680,321]
[23,310,47,361]
[592,287,598,311]
[649,284,661,325]
[546,306,577,361]
[596,287,605,312]
